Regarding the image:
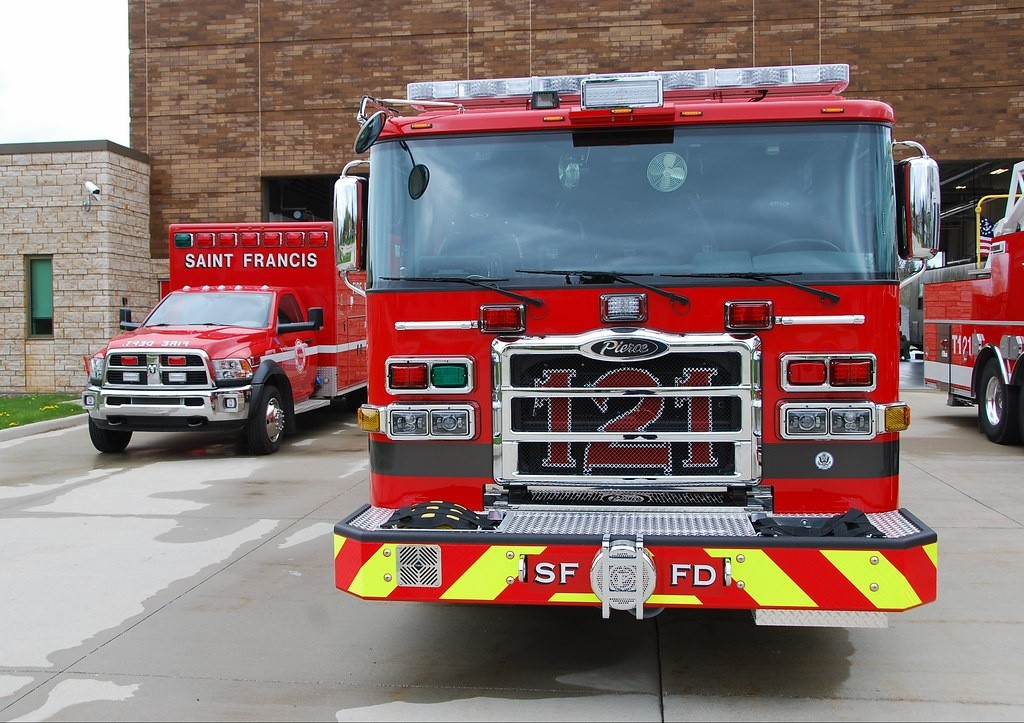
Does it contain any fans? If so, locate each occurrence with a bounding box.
[557,152,586,193]
[645,151,687,193]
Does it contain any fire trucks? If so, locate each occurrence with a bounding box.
[920,158,1024,448]
[330,62,941,630]
[84,221,365,458]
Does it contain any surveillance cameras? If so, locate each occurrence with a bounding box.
[81,181,101,213]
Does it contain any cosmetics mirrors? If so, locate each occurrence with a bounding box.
[895,154,942,261]
[331,175,369,273]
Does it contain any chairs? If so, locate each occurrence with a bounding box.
[437,227,521,278]
[264,308,291,324]
[738,190,838,255]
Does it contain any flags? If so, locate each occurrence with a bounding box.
[979,214,997,262]
